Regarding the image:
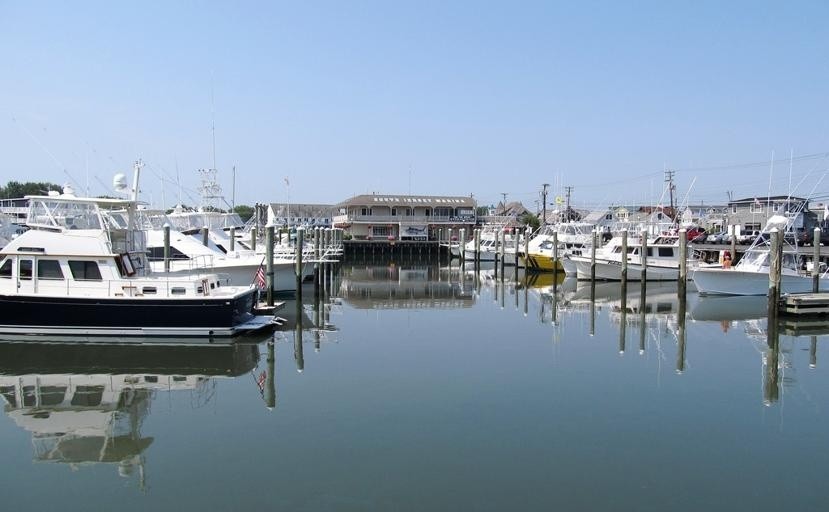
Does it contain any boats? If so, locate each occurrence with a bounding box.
[0,339,261,491]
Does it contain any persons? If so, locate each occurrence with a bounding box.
[722,256,732,268]
[799,234,813,265]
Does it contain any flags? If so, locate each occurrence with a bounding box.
[258,369,267,398]
[255,264,267,290]
